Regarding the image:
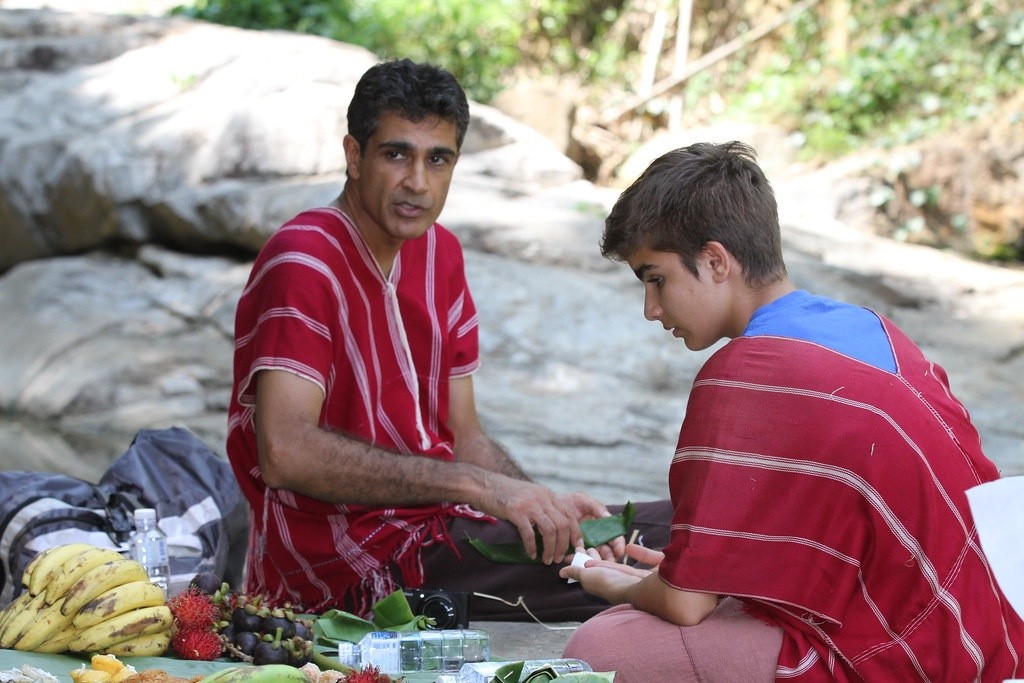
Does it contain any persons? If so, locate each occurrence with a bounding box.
[561,140,1024,683]
[226,59,673,621]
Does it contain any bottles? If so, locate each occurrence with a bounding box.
[129,508,170,602]
[438,659,594,683]
[337,629,491,675]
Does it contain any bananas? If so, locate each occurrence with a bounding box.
[0,543,174,658]
